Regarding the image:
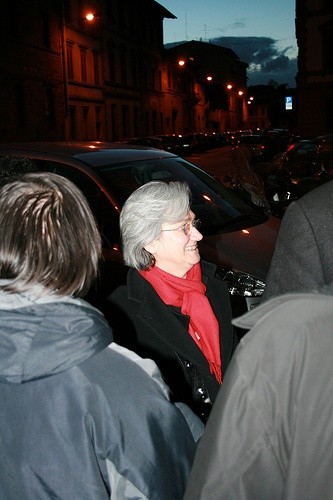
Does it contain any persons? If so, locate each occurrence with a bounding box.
[1,172,198,500]
[257,181,332,304]
[180,291,333,500]
[105,180,240,427]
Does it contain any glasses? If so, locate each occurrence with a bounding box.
[162,219,200,236]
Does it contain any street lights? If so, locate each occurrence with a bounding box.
[200,67,218,90]
[224,76,234,94]
[166,55,189,143]
[62,4,97,142]
[238,83,247,97]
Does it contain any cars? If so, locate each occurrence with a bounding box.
[95,128,316,170]
[0,140,282,299]
